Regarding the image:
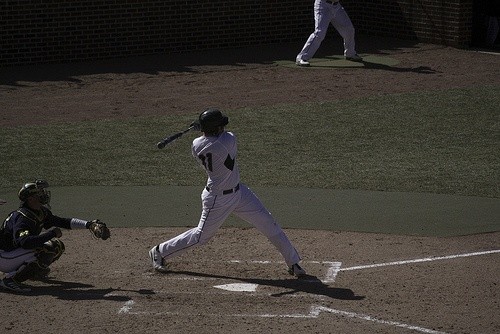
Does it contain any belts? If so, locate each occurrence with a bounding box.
[206,183,239,194]
[326,0,338,5]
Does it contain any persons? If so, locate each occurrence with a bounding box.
[0,179,111,294]
[148,109,306,277]
[296,0,363,66]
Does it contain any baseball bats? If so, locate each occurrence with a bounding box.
[157,125,194,149]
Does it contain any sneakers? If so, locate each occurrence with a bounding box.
[346,54,362,61]
[149,245,164,271]
[296,59,310,65]
[0,274,31,292]
[38,267,51,275]
[288,263,306,276]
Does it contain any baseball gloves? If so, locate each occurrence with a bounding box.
[89,219,111,240]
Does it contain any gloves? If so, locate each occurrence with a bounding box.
[49,227,62,238]
[190,120,201,129]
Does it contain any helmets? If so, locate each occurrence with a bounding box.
[200,110,229,131]
[18,183,42,208]
